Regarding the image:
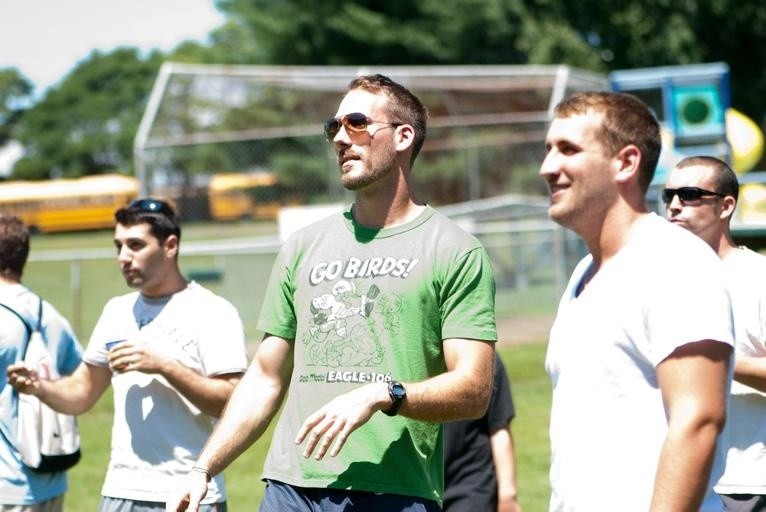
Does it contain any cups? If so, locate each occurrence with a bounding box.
[103,340,127,374]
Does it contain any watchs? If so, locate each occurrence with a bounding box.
[380,380,407,416]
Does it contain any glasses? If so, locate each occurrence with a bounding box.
[662,187,724,204]
[323,113,398,138]
[128,199,176,226]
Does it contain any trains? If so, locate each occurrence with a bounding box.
[0,170,302,235]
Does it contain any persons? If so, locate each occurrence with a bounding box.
[660,155,766,512]
[440,347,522,511]
[536,90,739,512]
[0,212,83,512]
[4,192,250,512]
[173,72,498,511]
